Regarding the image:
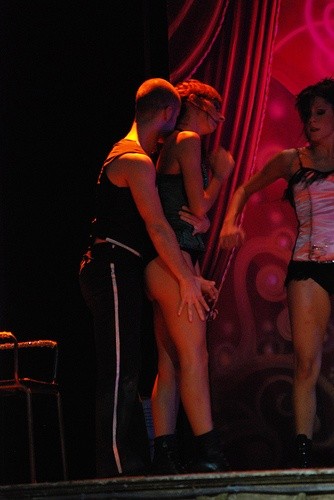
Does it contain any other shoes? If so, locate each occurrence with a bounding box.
[188,429,234,473]
[88,461,152,478]
[292,434,313,468]
[152,434,190,473]
[124,455,154,476]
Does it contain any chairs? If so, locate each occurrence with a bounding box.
[0,331,69,485]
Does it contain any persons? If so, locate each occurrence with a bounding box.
[218,76,333,472]
[76,78,218,478]
[141,81,225,478]
